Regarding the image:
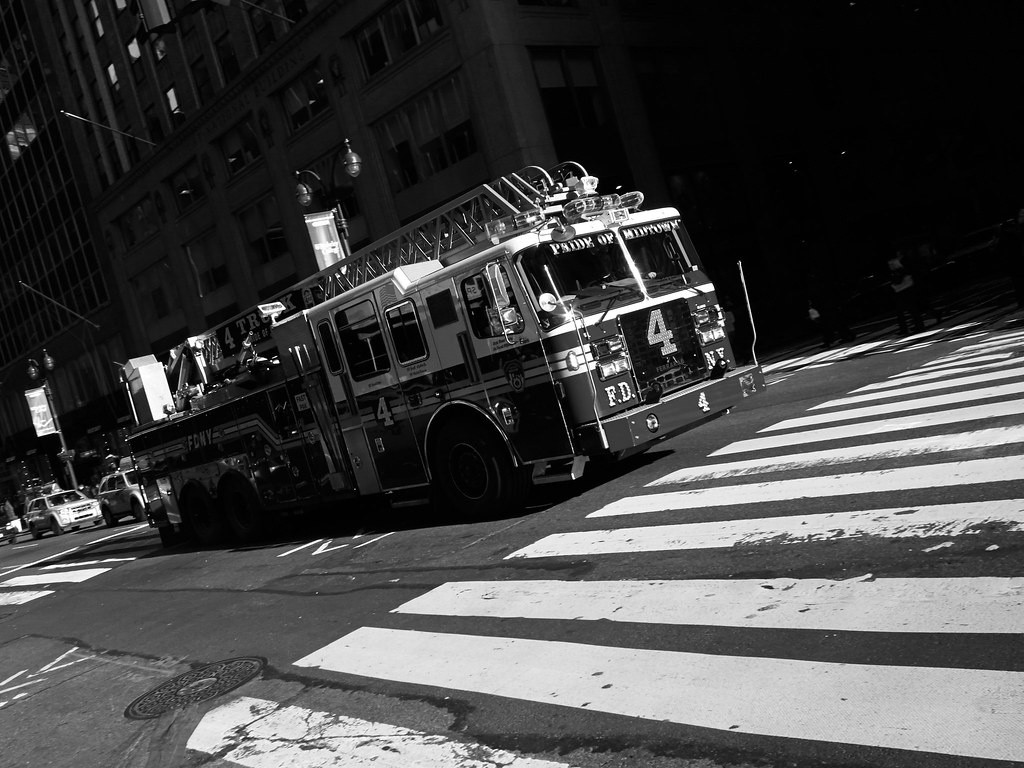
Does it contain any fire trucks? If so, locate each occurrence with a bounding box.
[110,160,767,552]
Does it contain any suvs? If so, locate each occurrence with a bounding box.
[97,468,150,529]
[26,489,104,541]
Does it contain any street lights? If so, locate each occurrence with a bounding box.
[292,136,363,274]
[24,348,78,490]
